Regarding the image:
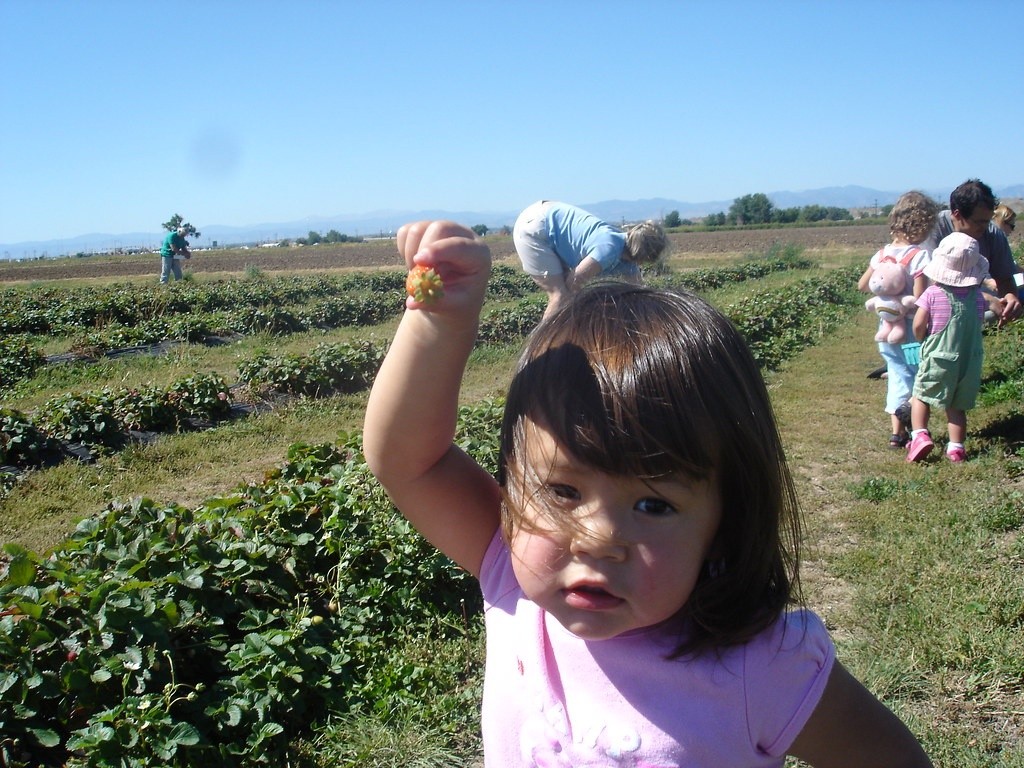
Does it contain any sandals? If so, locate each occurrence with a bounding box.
[887,434,910,451]
[895,402,932,438]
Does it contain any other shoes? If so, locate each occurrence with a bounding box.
[867,364,888,380]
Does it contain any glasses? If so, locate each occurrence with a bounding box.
[1005,221,1016,231]
[966,215,990,228]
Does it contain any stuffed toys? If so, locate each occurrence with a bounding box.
[863,262,918,345]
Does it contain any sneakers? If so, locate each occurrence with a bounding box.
[946,447,967,464]
[906,432,934,464]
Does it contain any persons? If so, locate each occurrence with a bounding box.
[512,199,669,323]
[160,228,191,285]
[857,177,1024,465]
[358,218,931,768]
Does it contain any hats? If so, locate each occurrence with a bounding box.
[922,232,989,287]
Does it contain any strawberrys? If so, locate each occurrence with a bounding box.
[405,264,447,304]
[67,650,77,662]
